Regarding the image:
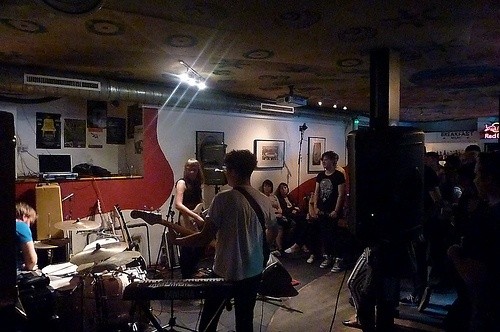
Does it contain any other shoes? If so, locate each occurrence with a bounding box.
[319,254,331,268]
[331,257,345,272]
[285,243,300,256]
[307,255,316,264]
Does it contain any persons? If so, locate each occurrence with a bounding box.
[285,192,316,263]
[277,183,309,253]
[175,158,205,280]
[342,144,500,332]
[262,180,290,253]
[15,203,38,274]
[167,150,278,332]
[314,151,346,271]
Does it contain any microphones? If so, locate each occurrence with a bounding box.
[169,193,175,213]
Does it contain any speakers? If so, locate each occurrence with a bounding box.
[0,110,19,305]
[201,143,228,186]
[347,128,424,238]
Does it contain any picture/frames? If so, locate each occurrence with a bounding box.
[196,130,224,161]
[308,137,326,172]
[87,223,151,266]
[254,140,285,169]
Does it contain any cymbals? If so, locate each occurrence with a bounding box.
[69,242,128,266]
[76,251,141,273]
[54,220,102,232]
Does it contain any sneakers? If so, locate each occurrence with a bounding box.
[399,285,432,312]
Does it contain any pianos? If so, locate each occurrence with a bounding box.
[122,278,232,332]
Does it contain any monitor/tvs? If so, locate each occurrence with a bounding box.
[39,155,72,174]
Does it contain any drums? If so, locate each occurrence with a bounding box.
[56,267,145,331]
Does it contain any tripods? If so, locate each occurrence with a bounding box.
[152,211,198,332]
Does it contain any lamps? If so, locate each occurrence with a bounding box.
[180,61,207,90]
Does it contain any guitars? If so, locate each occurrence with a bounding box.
[97,199,108,230]
[114,204,147,270]
[180,203,210,232]
[107,212,116,234]
[131,205,216,256]
